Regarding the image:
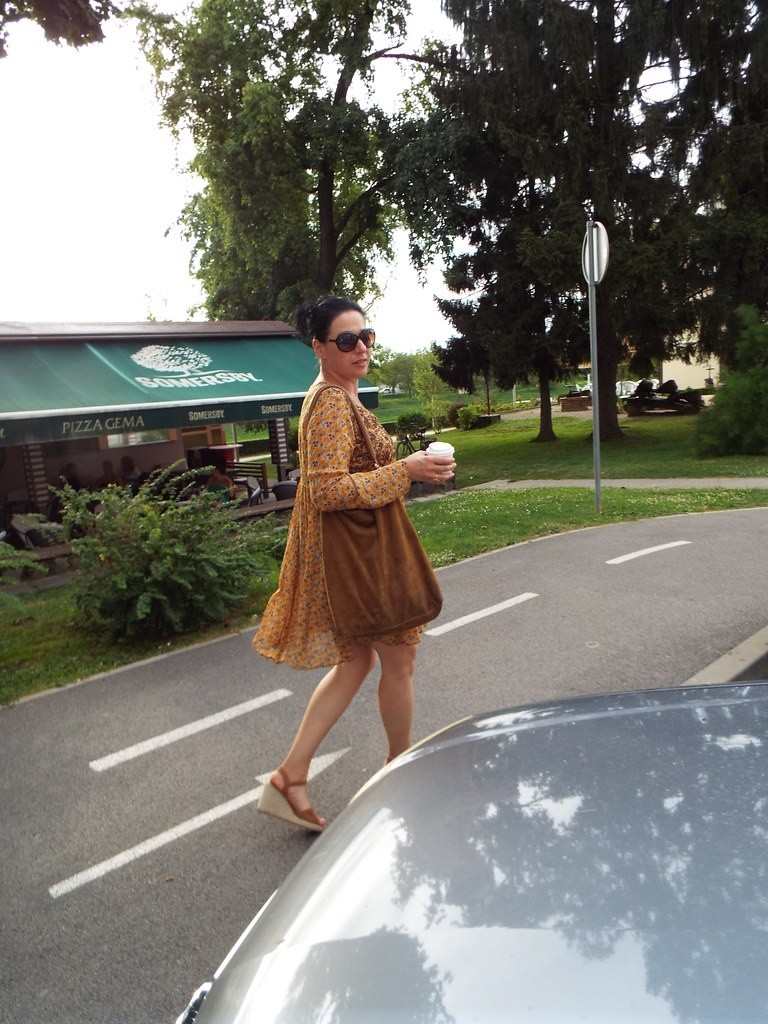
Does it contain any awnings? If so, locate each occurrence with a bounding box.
[0,335,380,448]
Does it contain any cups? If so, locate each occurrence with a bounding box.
[426,442,456,475]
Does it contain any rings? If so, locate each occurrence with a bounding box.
[436,474,440,481]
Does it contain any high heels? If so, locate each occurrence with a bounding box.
[256,766,325,831]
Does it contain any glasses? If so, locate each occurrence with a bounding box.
[322,328,375,353]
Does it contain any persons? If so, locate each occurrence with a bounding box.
[252,297,456,831]
[206,465,235,498]
[57,456,141,492]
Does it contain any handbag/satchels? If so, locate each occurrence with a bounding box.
[303,384,443,638]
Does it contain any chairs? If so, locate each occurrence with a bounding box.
[11,512,65,549]
[233,477,300,510]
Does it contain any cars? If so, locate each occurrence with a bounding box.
[378,388,396,394]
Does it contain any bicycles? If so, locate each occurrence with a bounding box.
[394,422,434,462]
